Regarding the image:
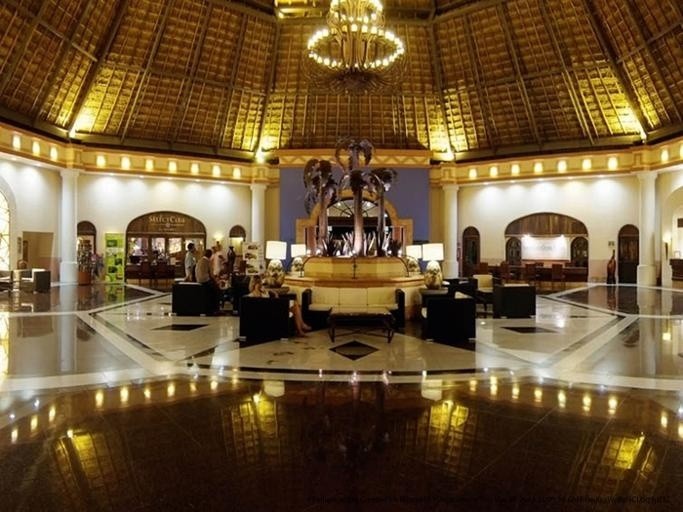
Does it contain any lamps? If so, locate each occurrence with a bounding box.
[299,0,411,100]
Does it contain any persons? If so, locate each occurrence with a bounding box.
[184,243,236,314]
[248,274,312,337]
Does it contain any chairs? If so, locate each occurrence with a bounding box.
[498,261,567,291]
[443,271,536,320]
[6,267,51,295]
[379,289,407,343]
[172,270,336,346]
